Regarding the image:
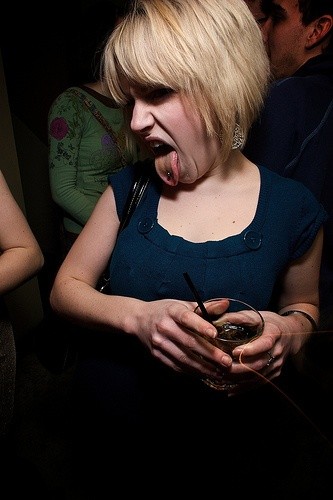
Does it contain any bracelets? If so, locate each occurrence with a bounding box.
[278,310,317,337]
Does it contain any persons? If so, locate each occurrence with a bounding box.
[242,0,333,500]
[0,168,44,438]
[50,0,329,500]
[48,48,135,383]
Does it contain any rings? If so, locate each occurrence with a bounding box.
[265,353,274,366]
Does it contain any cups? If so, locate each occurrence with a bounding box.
[194,298,265,391]
